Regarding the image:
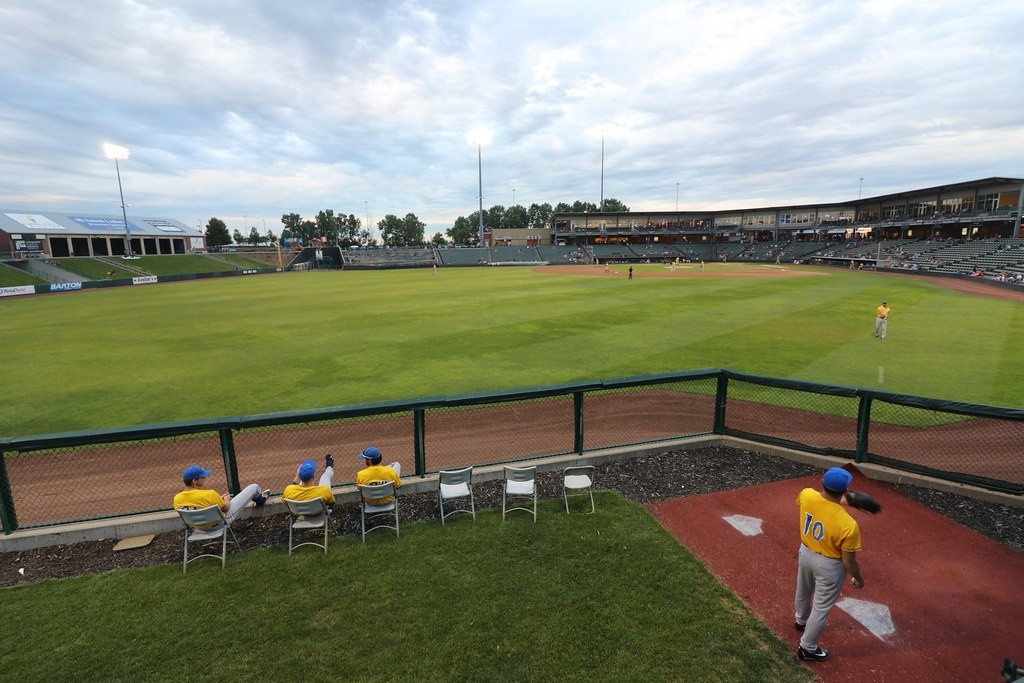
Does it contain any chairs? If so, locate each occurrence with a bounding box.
[561,465,595,515]
[436,466,474,527]
[358,480,399,542]
[283,497,338,557]
[175,504,242,574]
[501,466,537,523]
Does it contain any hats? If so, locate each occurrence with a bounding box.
[183,465,212,480]
[299,459,316,483]
[823,467,852,493]
[357,447,380,459]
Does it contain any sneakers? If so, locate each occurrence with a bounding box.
[795,622,806,630]
[797,646,830,661]
[262,489,270,503]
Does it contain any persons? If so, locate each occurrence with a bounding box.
[356,447,401,506]
[875,301,890,339]
[432,265,437,274]
[477,259,486,265]
[993,271,1023,283]
[628,266,633,280]
[973,264,978,271]
[564,251,585,262]
[890,246,944,270]
[852,207,960,225]
[517,249,525,254]
[924,232,1008,243]
[280,454,336,516]
[110,270,116,276]
[795,467,865,660]
[594,240,705,273]
[811,232,879,271]
[174,465,270,531]
[718,239,803,264]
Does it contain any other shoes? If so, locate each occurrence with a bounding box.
[326,454,334,468]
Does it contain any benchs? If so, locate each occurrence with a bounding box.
[337,225,1024,292]
